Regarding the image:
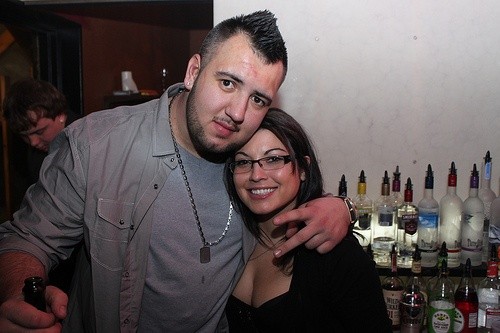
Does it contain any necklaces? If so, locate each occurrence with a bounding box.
[248,235,286,263]
[169,89,234,264]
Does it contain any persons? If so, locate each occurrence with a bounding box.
[223,108,393,333]
[0,9,358,333]
[5,79,92,223]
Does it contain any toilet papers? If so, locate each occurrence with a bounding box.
[121,70,132,91]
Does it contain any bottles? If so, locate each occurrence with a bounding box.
[23,276,46,313]
[338,150,500,333]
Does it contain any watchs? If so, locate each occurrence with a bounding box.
[334,195,358,230]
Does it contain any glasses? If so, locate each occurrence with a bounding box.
[227,155,298,174]
